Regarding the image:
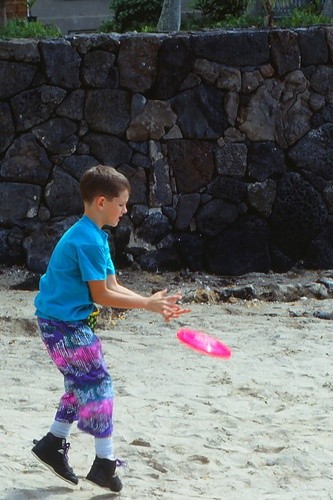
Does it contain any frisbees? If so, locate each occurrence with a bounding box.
[178,330,232,361]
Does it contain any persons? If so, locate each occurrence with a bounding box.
[30,164,191,493]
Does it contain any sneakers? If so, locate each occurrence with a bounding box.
[85,461,122,493]
[31,436,79,487]
